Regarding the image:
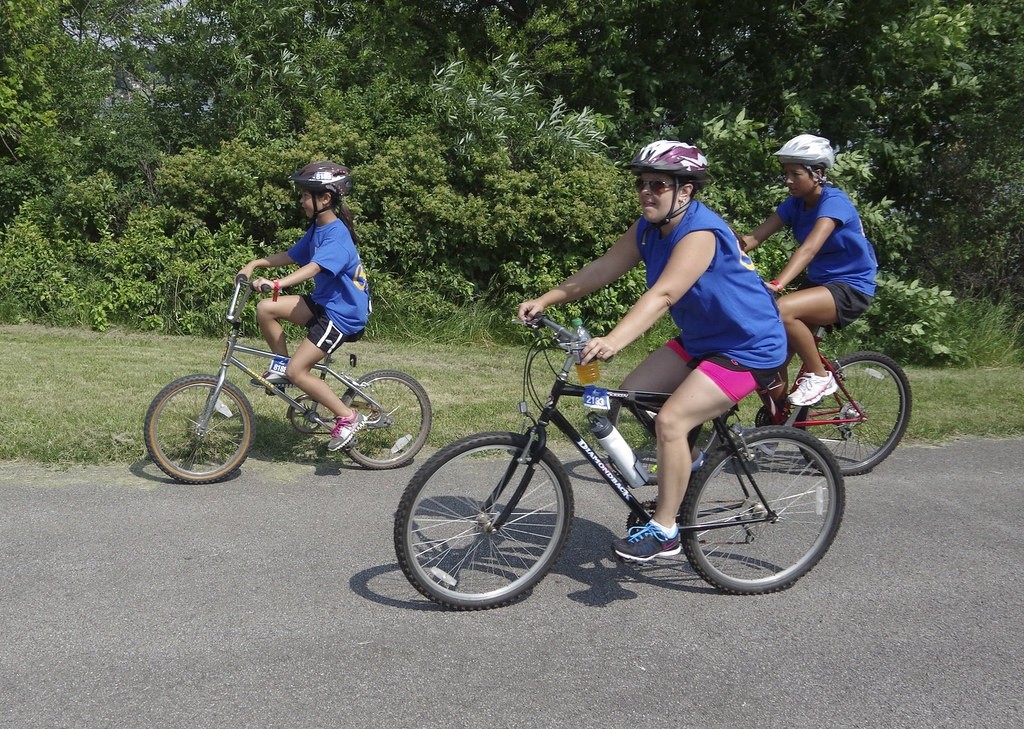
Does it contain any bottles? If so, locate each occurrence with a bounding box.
[586,410,650,489]
[570,317,601,385]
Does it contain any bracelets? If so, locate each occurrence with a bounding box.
[272,279,279,302]
[769,280,784,292]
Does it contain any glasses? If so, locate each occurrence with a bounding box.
[635,179,684,195]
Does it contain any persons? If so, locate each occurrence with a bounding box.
[235,160,372,452]
[742,134,878,406]
[517,140,788,562]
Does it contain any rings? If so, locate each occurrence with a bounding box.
[599,351,604,355]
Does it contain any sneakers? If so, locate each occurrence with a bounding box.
[691,449,721,478]
[787,371,839,406]
[249,370,298,388]
[327,409,367,451]
[611,521,681,561]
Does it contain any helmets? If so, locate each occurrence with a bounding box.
[287,161,353,197]
[623,139,707,190]
[773,134,834,171]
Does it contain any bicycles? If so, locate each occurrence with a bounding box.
[753,320,915,478]
[142,273,432,484]
[391,316,848,611]
